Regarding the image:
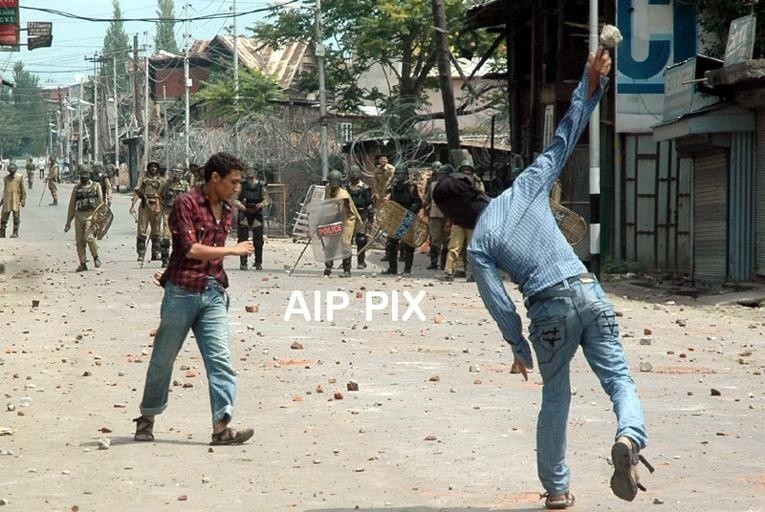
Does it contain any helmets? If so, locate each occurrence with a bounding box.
[327,158,477,185]
[79,161,257,180]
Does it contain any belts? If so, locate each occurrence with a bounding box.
[524,272,595,310]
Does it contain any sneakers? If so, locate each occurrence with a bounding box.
[610,435,643,500]
[73,252,170,271]
[0,232,19,238]
[542,491,575,509]
[240,260,264,271]
[322,261,456,283]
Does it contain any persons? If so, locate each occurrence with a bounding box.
[324,154,485,282]
[132,152,255,446]
[0,162,27,238]
[25,154,205,273]
[432,44,654,508]
[232,160,272,270]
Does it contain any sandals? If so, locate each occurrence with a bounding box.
[209,427,254,446]
[132,415,156,442]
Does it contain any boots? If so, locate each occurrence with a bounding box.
[48,199,59,205]
